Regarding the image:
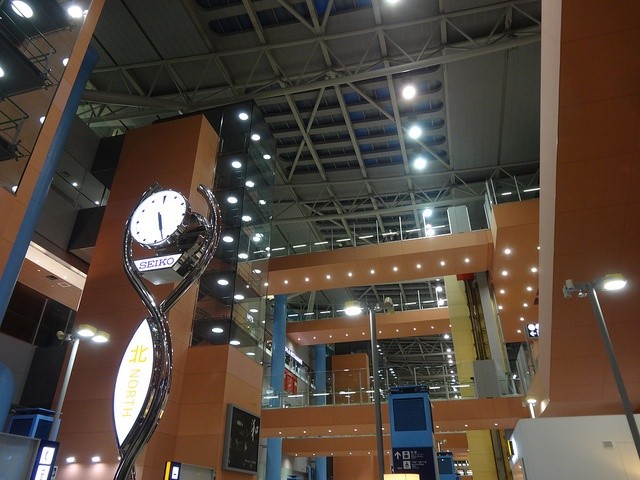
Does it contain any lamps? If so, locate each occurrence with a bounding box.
[602,272,628,292]
[77,324,110,344]
[344,301,362,317]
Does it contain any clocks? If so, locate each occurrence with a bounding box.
[129,189,187,245]
